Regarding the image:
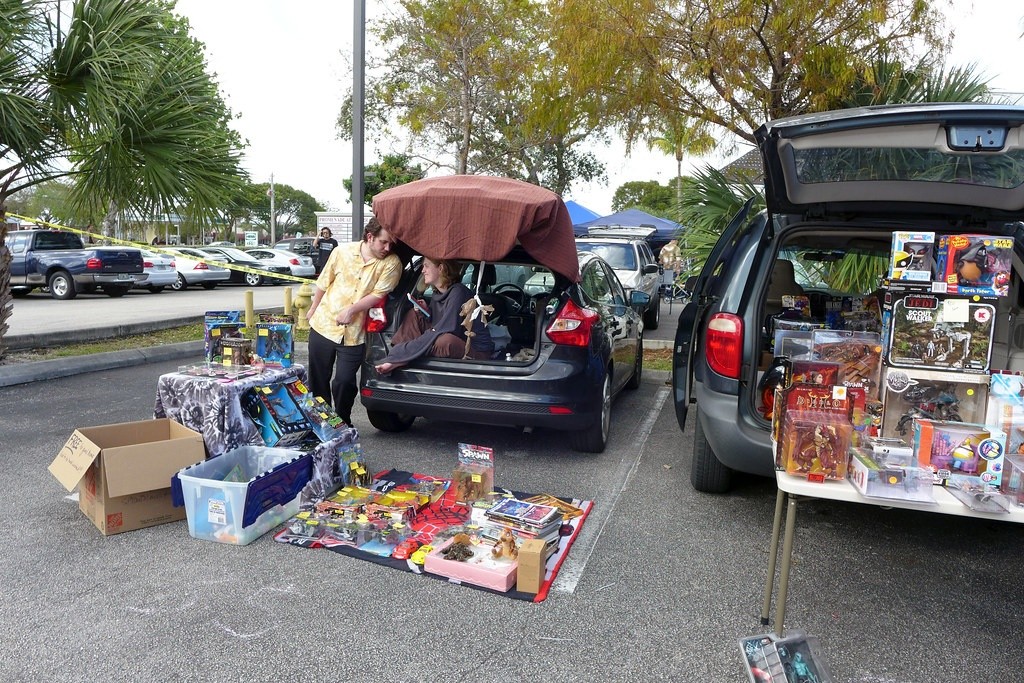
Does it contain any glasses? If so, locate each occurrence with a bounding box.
[323,231,330,235]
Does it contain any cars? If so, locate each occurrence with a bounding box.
[136,250,178,294]
[356,175,651,460]
[244,247,317,278]
[198,246,292,288]
[158,247,231,291]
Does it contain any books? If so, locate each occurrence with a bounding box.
[406,293,432,319]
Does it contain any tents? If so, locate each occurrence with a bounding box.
[572,207,690,288]
[562,200,604,228]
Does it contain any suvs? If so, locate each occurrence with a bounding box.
[269,237,323,272]
[575,223,665,327]
[666,101,1022,532]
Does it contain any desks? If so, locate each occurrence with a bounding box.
[159,363,305,394]
[761,440,1024,638]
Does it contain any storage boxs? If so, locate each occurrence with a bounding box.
[171,444,314,547]
[48,417,206,536]
[515,539,548,594]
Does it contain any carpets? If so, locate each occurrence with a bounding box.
[274,468,594,602]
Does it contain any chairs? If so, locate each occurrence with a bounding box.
[471,264,522,341]
[763,259,804,326]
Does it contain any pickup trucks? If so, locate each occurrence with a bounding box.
[5,229,149,300]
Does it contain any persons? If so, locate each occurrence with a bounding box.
[658,238,683,294]
[305,217,403,427]
[311,227,338,278]
[372,254,496,375]
